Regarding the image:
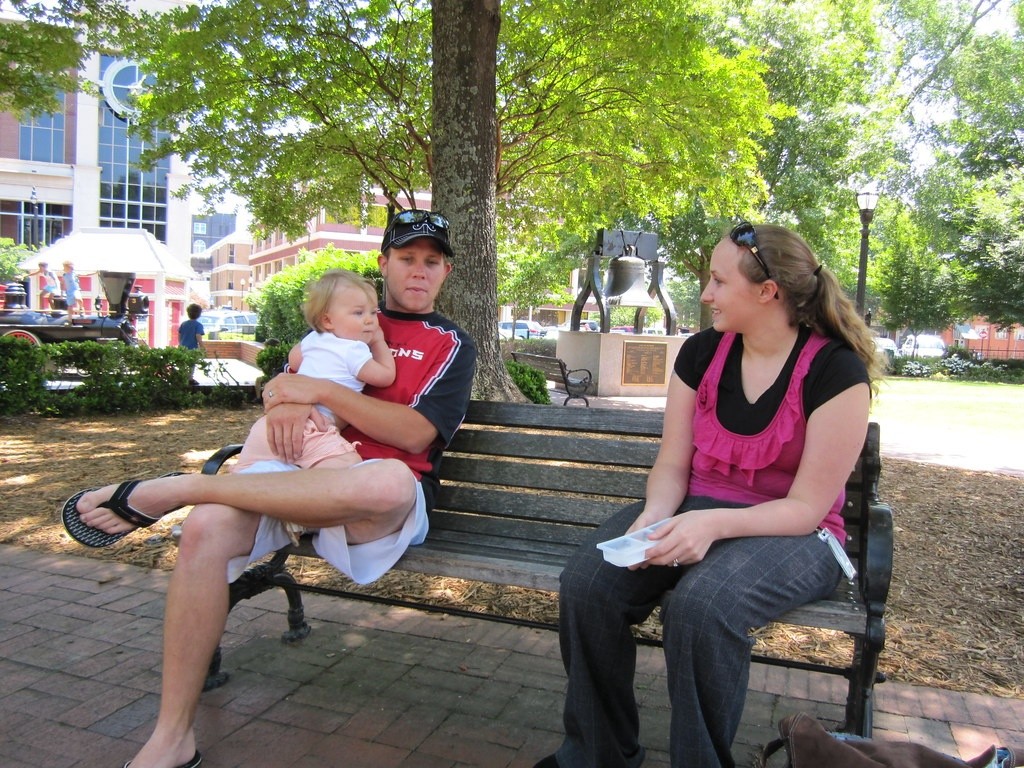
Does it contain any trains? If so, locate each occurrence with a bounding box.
[0,264,151,377]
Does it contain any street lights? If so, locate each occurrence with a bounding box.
[240,279,245,309]
[856,191,879,321]
[30,187,38,250]
[248,277,253,292]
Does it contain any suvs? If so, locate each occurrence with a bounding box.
[558,320,601,332]
[498,321,546,341]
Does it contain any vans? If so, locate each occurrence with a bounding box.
[198,310,249,333]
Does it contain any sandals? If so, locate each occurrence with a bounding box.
[283,519,305,547]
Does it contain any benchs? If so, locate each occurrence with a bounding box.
[198,399,894,739]
[511,352,595,407]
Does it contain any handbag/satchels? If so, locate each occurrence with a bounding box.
[761,712,1024,768]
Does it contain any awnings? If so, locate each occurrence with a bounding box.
[953,322,982,339]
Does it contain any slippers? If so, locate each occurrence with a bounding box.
[61,471,189,549]
[122,749,201,768]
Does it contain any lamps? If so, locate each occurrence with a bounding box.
[94,295,102,315]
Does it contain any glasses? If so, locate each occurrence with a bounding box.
[385,211,450,240]
[730,221,779,300]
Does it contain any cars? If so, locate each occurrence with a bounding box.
[872,337,901,358]
[499,327,523,341]
[610,326,661,334]
[902,335,945,358]
[244,313,259,326]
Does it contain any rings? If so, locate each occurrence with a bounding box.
[268,391,273,397]
[673,558,679,567]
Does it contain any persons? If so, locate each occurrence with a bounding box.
[28,261,60,310]
[533,222,893,768]
[177,304,206,385]
[60,260,98,320]
[230,268,396,547]
[60,209,477,768]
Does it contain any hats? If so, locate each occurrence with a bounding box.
[381,210,455,257]
